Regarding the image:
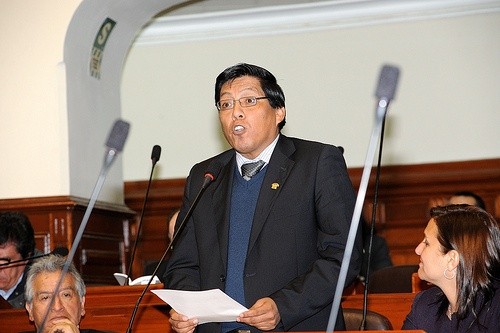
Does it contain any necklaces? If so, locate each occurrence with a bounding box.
[448,305,453,320]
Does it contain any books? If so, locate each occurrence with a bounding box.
[113,273,160,286]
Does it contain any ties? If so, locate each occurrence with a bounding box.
[241,160,266,180]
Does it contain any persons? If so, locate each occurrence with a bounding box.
[354,192,500,333]
[141,209,179,276]
[161,61,367,333]
[0,209,37,312]
[24,254,111,333]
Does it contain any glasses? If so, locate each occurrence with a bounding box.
[0,257,11,267]
[214,96,269,111]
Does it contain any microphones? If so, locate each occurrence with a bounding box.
[327,65,400,331]
[126,163,222,333]
[126,145,162,285]
[0,247,68,266]
[37,121,130,333]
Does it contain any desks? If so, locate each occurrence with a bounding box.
[85,287,422,333]
[0,307,37,333]
